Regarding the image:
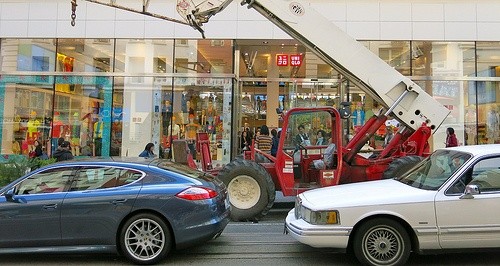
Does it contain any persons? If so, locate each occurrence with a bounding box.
[53,137,65,158]
[353,102,365,132]
[71,112,82,155]
[443,127,458,148]
[167,120,180,141]
[271,128,279,158]
[185,114,199,144]
[295,124,310,148]
[57,141,74,161]
[25,111,40,151]
[138,143,157,158]
[32,139,42,159]
[163,100,172,134]
[183,99,193,113]
[315,130,328,146]
[241,131,250,152]
[254,125,272,164]
[94,115,103,156]
[49,111,64,153]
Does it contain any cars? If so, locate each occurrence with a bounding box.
[286,144,500,266]
[0,158,234,266]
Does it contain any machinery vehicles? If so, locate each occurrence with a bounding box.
[70,1,452,222]
